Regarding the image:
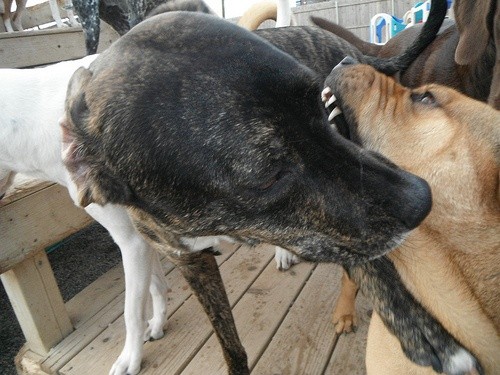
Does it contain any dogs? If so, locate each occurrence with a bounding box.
[0,0,500,375]
